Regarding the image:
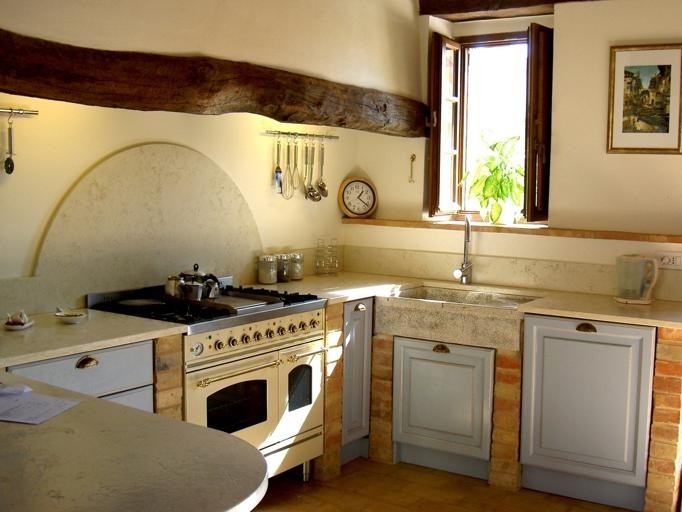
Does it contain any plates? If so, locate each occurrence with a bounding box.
[5,319,35,330]
[53,312,86,324]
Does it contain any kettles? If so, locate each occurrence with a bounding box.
[613,254,658,304]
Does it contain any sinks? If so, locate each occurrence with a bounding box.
[373,285,544,353]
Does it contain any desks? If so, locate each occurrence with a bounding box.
[0,369,270,512]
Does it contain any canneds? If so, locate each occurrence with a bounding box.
[258,254,304,284]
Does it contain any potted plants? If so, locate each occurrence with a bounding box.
[456,134,525,225]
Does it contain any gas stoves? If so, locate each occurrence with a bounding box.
[84,283,325,365]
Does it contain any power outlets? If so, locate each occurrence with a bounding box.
[657,250,682,271]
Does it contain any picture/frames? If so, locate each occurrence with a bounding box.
[606,42,681,155]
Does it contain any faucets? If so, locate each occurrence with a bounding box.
[460,213,472,284]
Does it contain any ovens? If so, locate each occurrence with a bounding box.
[182,332,325,482]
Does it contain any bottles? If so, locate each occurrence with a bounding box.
[316,238,341,275]
[259,252,304,284]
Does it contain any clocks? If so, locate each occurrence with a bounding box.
[338,176,379,218]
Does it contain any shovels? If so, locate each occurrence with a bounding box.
[303,147,308,199]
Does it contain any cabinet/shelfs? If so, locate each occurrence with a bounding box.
[342,298,374,465]
[393,334,496,485]
[520,312,657,511]
[6,340,156,413]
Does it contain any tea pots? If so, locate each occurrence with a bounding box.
[166,265,221,301]
